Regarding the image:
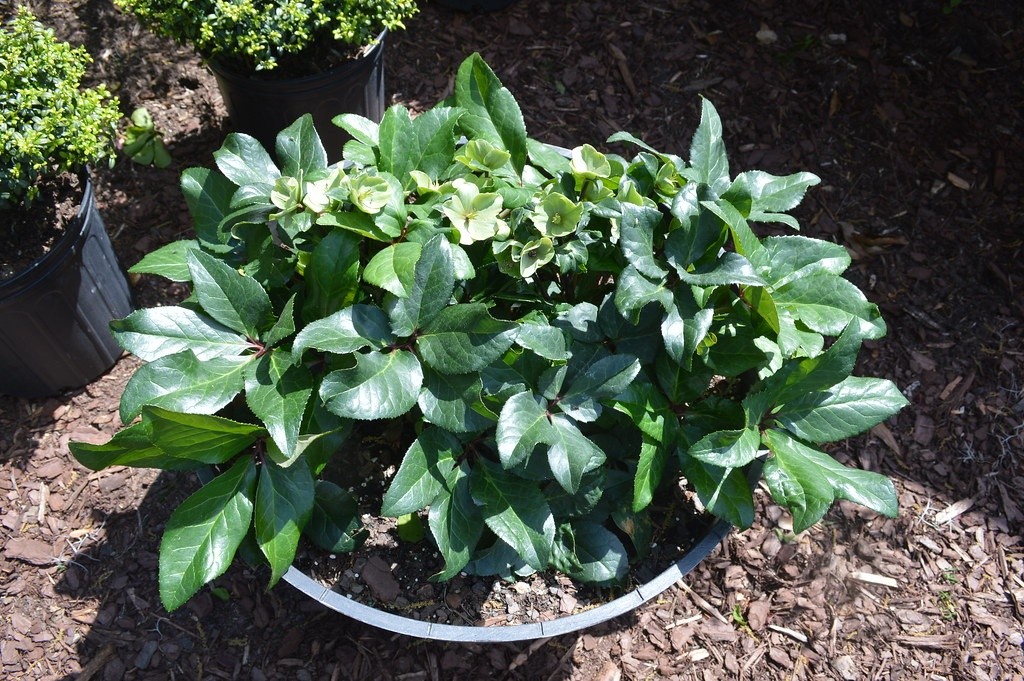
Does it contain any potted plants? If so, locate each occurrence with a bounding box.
[0,5,137,403]
[66,53,912,645]
[112,0,420,173]
